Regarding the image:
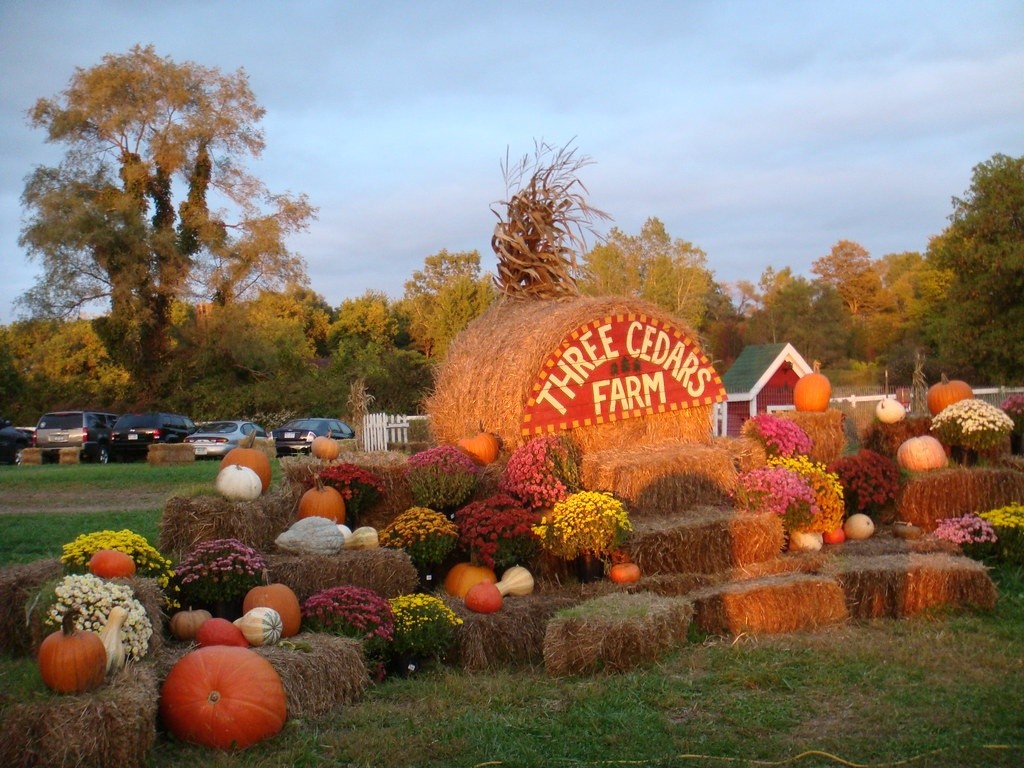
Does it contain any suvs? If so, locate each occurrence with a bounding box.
[109,412,197,461]
[32,410,118,465]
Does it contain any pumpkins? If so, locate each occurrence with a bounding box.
[793,363,831,412]
[610,562,640,584]
[876,398,906,424]
[214,428,378,556]
[158,567,302,754]
[790,514,874,551]
[455,431,499,466]
[927,372,973,417]
[445,553,534,613]
[38,605,129,697]
[893,521,921,539]
[88,549,136,579]
[897,434,947,473]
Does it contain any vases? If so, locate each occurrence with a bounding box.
[1009,432,1024,458]
[950,444,978,469]
[208,597,242,623]
[415,564,439,595]
[573,546,606,584]
[389,649,422,680]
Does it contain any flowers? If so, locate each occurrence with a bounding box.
[385,592,461,654]
[392,442,479,511]
[62,524,183,611]
[454,494,541,568]
[305,462,387,528]
[535,486,635,562]
[494,435,583,510]
[299,582,393,641]
[927,393,1024,560]
[725,414,900,533]
[43,574,154,668]
[376,506,459,571]
[176,536,272,601]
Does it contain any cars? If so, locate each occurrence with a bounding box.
[183,420,268,460]
[268,418,355,457]
[0,420,36,464]
